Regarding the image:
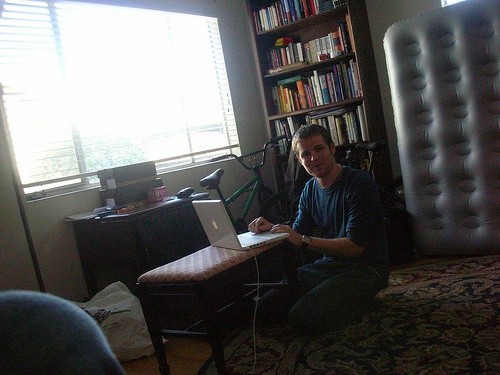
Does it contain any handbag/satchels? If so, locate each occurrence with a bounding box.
[69,281,168,362]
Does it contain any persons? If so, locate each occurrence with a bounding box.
[247,122,389,335]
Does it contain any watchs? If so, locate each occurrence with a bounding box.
[301,234,312,250]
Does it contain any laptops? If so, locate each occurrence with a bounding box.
[192,199,289,250]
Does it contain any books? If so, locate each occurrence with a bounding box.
[262,23,353,74]
[270,105,368,155]
[270,59,361,118]
[251,0,346,34]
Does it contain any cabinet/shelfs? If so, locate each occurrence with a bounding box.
[244,0,402,266]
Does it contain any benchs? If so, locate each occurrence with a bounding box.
[135,235,307,375]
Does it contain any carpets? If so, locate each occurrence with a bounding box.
[199,257,500,375]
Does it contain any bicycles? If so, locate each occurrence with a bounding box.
[199,134,286,234]
[257,138,406,255]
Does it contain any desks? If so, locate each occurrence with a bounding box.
[72,193,216,334]
